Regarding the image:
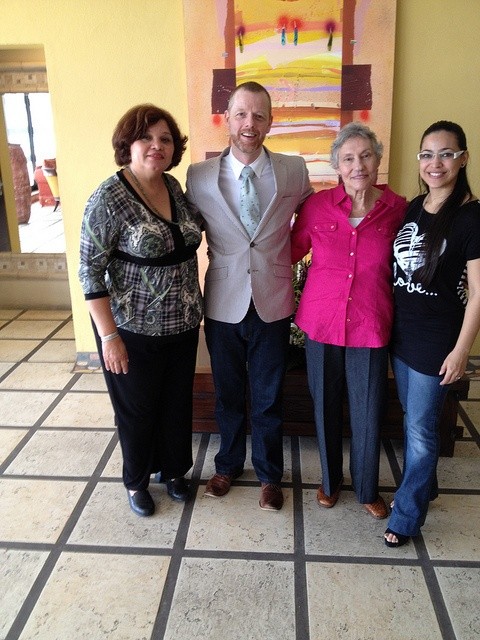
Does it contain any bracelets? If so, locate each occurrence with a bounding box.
[101,331,119,343]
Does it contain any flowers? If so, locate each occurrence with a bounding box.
[290,257,312,349]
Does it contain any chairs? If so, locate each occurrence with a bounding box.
[41,168,61,212]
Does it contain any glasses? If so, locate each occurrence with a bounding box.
[416,149,465,161]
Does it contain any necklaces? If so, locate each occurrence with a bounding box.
[126,166,166,220]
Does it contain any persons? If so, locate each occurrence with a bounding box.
[185,82,315,511]
[80,105,203,515]
[385,120,480,547]
[207,122,410,519]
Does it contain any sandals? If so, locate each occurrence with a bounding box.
[383,528,410,547]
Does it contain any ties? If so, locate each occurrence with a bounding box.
[240,165,261,239]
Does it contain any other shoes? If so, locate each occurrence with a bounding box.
[362,494,388,520]
[316,477,344,508]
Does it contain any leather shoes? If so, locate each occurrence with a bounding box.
[127,488,155,517]
[167,476,193,501]
[203,469,245,498]
[258,480,284,511]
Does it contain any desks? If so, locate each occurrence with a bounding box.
[192,325,470,441]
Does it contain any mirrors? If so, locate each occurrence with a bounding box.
[1,92,66,255]
